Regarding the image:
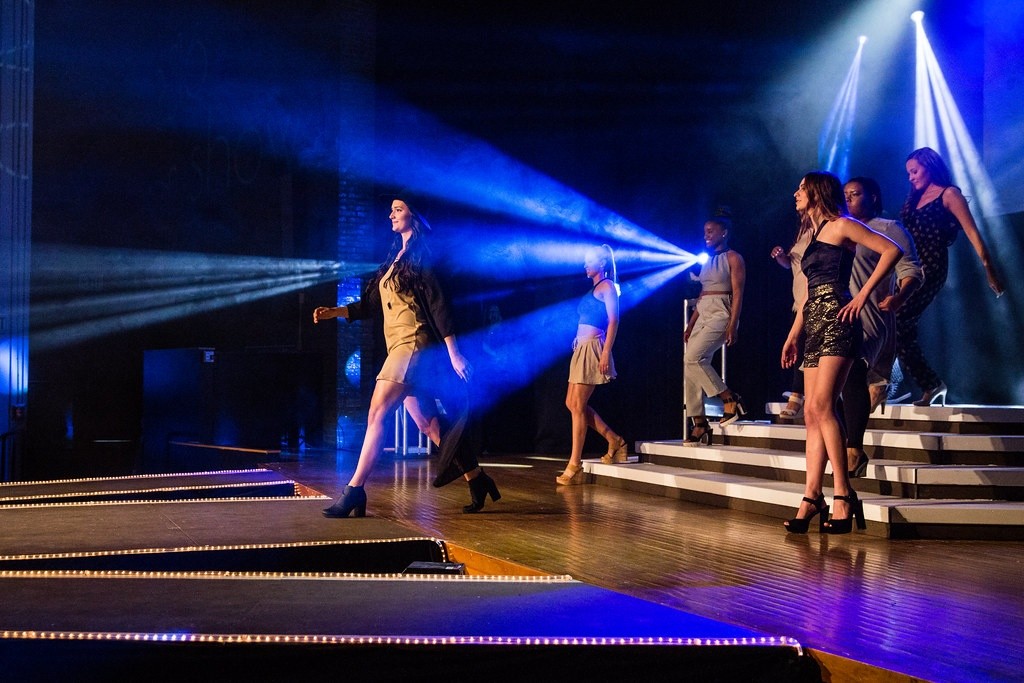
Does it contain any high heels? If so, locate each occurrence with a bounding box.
[783,492,831,533]
[869,392,887,415]
[913,381,950,407]
[462,466,502,513]
[555,461,585,485]
[823,488,867,534]
[718,391,748,428]
[601,435,628,464]
[780,396,804,421]
[831,451,869,480]
[321,484,367,519]
[683,420,714,447]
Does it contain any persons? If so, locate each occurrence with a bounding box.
[773,146,1006,534]
[553,244,628,484]
[682,214,746,447]
[312,194,502,520]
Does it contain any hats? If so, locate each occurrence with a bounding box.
[396,186,432,234]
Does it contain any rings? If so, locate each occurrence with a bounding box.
[607,366,609,367]
